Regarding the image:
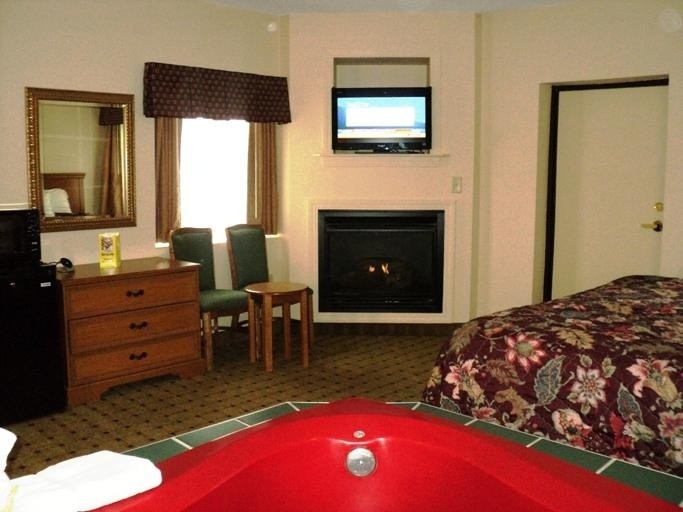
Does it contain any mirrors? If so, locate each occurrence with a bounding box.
[24,86,137,234]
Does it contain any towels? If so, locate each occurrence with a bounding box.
[0,427,163,512]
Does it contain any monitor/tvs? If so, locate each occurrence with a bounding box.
[331,86,431,153]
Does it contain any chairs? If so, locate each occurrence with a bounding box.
[166,223,314,371]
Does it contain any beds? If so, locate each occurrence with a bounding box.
[426,275,682,478]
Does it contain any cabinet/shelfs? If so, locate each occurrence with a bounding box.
[54,256,205,408]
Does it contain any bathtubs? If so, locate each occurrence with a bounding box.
[86,395,683,512]
